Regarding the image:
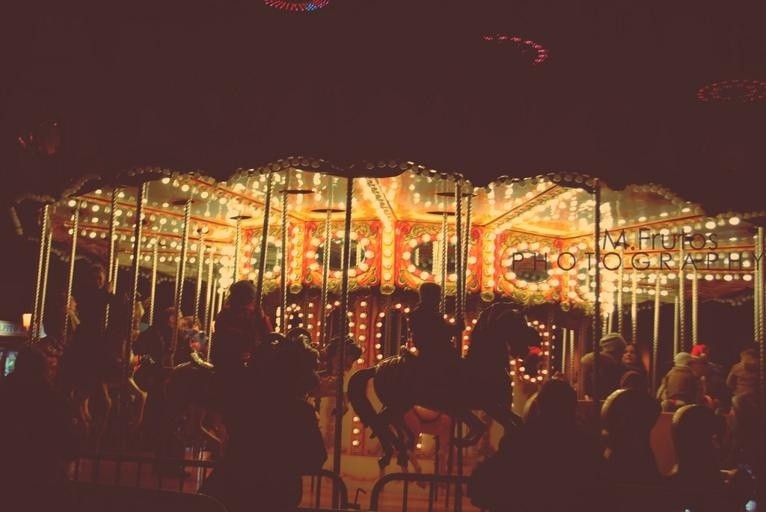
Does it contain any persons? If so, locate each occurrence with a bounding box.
[408,282,765,511]
[2,264,327,511]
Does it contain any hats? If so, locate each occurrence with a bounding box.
[674,352,690,365]
[691,344,709,359]
[600,332,626,352]
